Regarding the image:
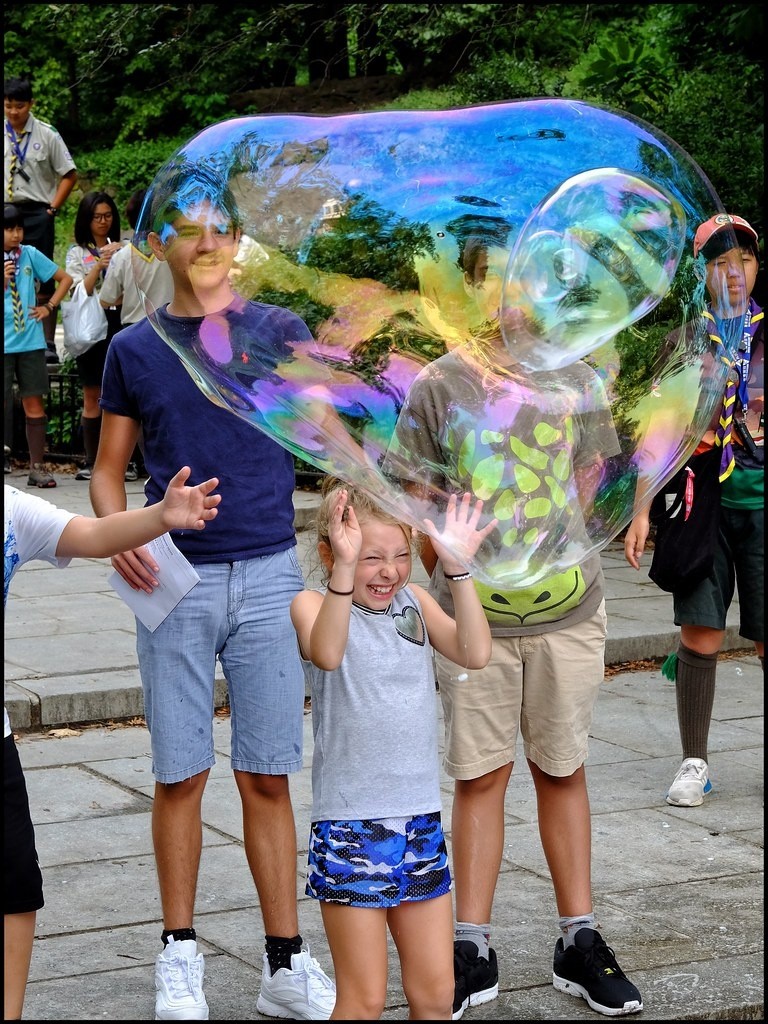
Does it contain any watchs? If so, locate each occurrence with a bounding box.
[48,206,60,216]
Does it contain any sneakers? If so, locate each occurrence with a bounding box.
[254,948,338,1020]
[451,940,499,1018]
[125,463,140,482]
[552,928,649,1015]
[153,940,211,1021]
[26,464,58,488]
[75,461,94,480]
[663,757,715,807]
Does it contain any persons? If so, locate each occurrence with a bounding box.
[624,214,764,807]
[381,227,643,1020]
[4,468,222,1020]
[89,168,398,1020]
[4,78,269,488]
[290,469,492,1020]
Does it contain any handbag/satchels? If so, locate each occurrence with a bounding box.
[647,450,726,590]
[62,280,110,358]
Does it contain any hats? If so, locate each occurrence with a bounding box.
[694,214,758,256]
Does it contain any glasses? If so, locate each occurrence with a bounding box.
[93,212,113,222]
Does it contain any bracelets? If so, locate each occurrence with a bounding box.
[327,582,355,596]
[43,304,53,316]
[48,300,56,311]
[443,570,473,581]
